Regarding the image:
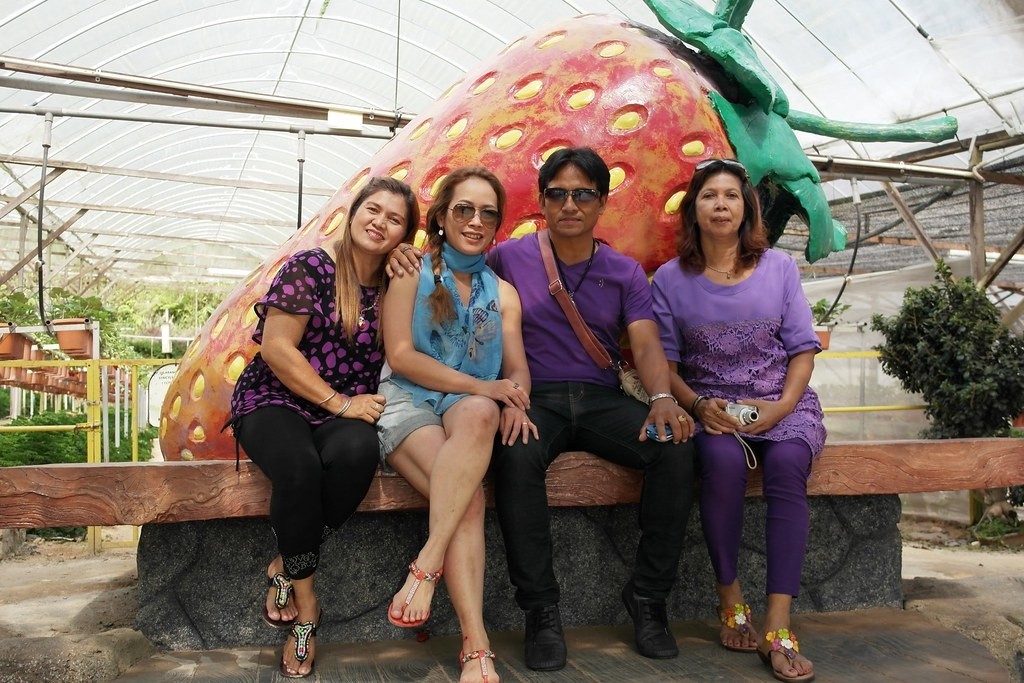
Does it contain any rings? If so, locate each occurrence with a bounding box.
[715,409,723,416]
[513,383,520,389]
[403,246,411,254]
[522,422,528,425]
[678,415,687,421]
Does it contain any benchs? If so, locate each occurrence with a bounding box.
[0,433,1024,529]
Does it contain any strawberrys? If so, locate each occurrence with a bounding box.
[158,0,959,459]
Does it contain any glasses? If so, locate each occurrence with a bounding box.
[544,187,600,202]
[446,203,502,229]
[695,159,746,171]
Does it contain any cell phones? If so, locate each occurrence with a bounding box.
[646,422,673,442]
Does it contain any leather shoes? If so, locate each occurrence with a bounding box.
[524,603,567,671]
[622,584,679,658]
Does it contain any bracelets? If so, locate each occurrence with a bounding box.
[692,396,709,412]
[334,397,350,416]
[338,400,352,417]
[318,390,337,407]
[649,393,677,406]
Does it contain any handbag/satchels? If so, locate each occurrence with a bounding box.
[617,358,650,406]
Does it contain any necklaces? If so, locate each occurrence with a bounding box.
[356,281,383,330]
[548,234,597,304]
[705,262,739,279]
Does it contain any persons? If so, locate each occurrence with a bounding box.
[230,177,421,677]
[375,166,539,683]
[651,158,827,683]
[386,149,696,672]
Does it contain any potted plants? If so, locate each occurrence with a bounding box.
[0,287,151,403]
[811,299,852,349]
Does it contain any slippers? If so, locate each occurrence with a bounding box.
[758,628,816,683]
[261,573,293,630]
[387,558,443,627]
[716,603,757,651]
[458,648,497,683]
[279,608,323,678]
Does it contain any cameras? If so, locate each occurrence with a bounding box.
[725,402,759,426]
